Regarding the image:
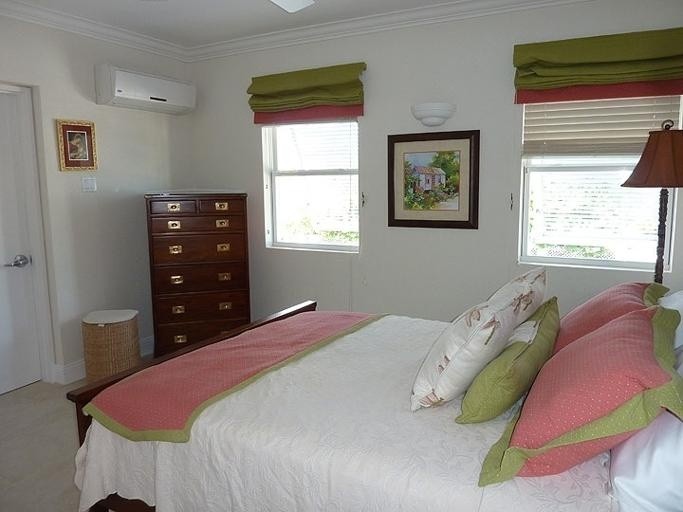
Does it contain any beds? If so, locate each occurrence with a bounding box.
[66,297,683,512]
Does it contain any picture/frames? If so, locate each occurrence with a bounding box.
[55,118,97,172]
[387,129,480,229]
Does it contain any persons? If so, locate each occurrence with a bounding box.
[69,134,84,159]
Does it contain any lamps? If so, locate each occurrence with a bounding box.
[618,119,682,285]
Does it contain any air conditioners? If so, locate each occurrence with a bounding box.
[93,61,196,116]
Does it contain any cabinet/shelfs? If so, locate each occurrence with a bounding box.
[144,192,251,357]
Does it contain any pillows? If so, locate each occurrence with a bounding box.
[409,296,520,412]
[490,266,546,328]
[454,294,561,424]
[478,305,682,486]
[545,283,670,354]
